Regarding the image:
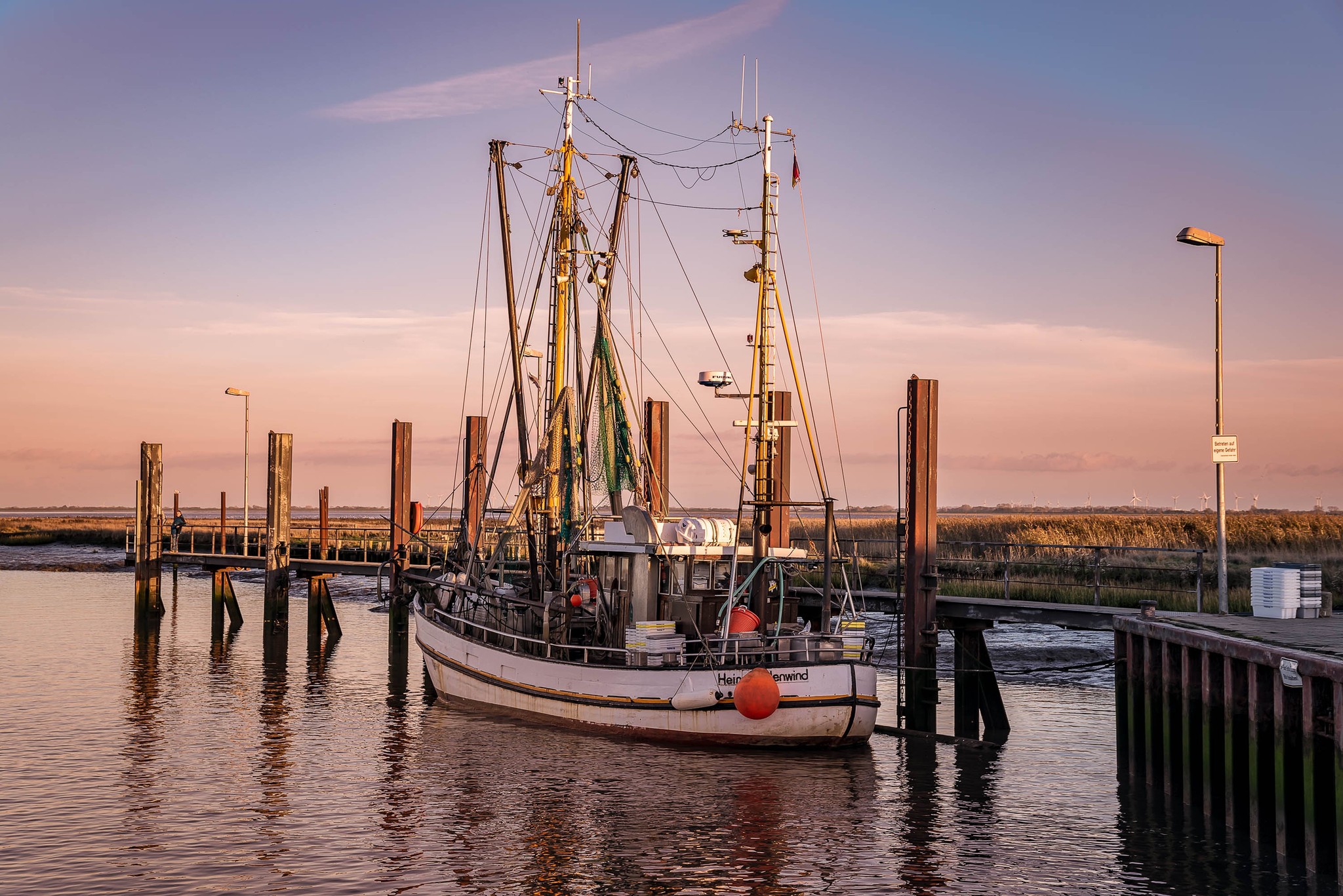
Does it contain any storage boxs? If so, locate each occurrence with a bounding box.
[626,620,686,666]
[840,620,865,661]
[718,631,758,648]
[470,607,498,645]
[1250,562,1322,619]
[724,646,773,665]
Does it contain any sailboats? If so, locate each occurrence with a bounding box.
[379,15,883,755]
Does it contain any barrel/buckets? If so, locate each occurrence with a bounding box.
[577,577,598,598]
[728,606,760,633]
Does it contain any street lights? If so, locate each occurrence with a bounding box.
[1175,226,1228,614]
[519,346,544,561]
[224,387,250,555]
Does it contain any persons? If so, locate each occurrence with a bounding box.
[169,510,186,553]
[161,510,167,527]
